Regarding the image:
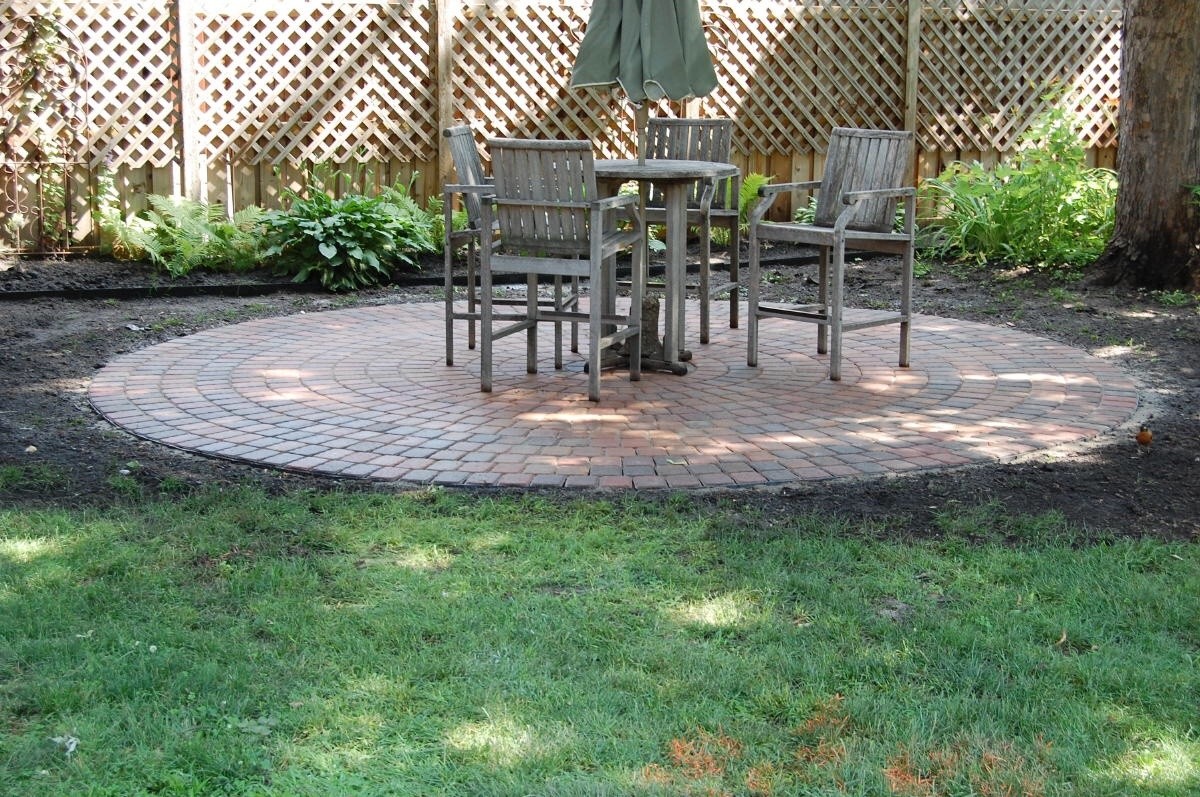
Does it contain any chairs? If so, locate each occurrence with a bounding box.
[442,118,916,401]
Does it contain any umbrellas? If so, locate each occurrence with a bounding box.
[569,0,719,376]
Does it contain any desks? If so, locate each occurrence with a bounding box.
[551,157,741,375]
